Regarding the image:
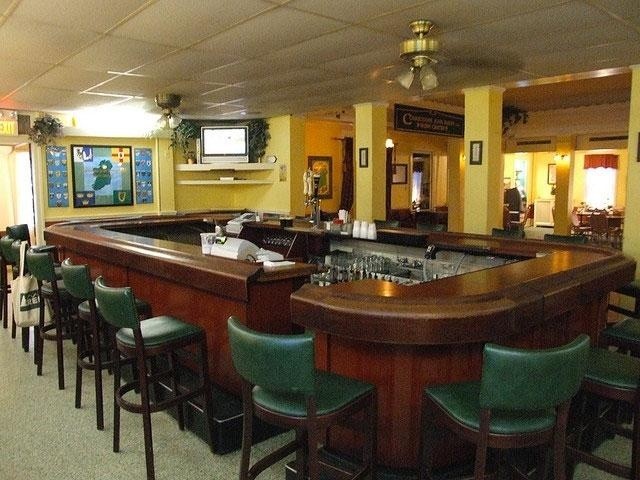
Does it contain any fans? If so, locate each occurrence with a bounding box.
[330,21,524,87]
[131,94,217,116]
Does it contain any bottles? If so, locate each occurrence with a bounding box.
[307,247,423,286]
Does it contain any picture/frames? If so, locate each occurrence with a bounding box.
[308,155,332,198]
[391,164,408,184]
[547,164,556,185]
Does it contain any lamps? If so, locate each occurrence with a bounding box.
[397,62,438,91]
[555,154,568,163]
[156,112,182,129]
[303,169,321,225]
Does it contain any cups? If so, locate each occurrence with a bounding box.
[360,221,368,238]
[353,221,361,238]
[368,224,377,240]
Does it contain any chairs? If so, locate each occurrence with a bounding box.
[422,335,590,480]
[10,241,30,346]
[591,214,619,248]
[559,341,640,479]
[94,275,212,479]
[572,213,591,239]
[374,220,401,228]
[544,234,586,243]
[1,236,16,327]
[26,245,96,389]
[417,224,447,232]
[228,318,377,480]
[608,277,639,324]
[491,229,524,240]
[64,257,131,408]
[588,315,640,454]
[508,206,532,236]
[7,225,33,250]
[32,246,63,364]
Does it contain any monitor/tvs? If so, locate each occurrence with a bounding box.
[240,213,257,221]
[201,126,249,164]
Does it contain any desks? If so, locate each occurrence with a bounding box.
[604,216,624,243]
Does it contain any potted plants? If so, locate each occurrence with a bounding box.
[170,121,200,164]
[501,106,528,137]
[245,121,271,163]
[29,115,61,146]
